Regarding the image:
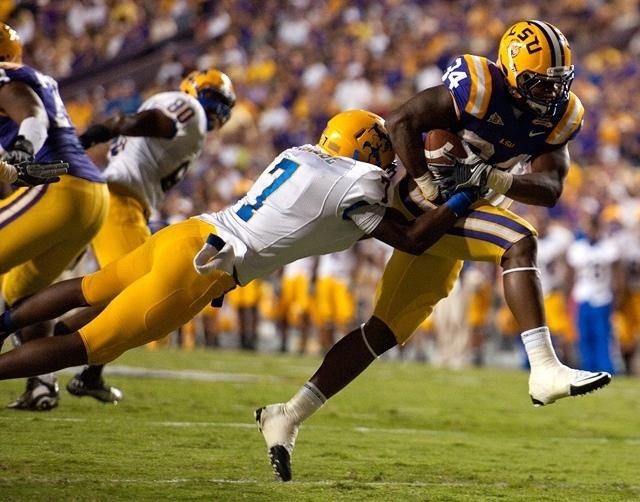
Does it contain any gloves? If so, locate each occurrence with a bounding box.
[428,141,514,216]
[12,162,68,186]
[4,136,37,168]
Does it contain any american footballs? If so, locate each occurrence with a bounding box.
[420,129,467,166]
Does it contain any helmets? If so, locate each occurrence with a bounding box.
[180,69,238,130]
[497,22,577,119]
[319,110,396,205]
[0,22,26,64]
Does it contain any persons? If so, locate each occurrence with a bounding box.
[0,20,111,412]
[251,19,613,482]
[2,1,640,376]
[9,66,238,404]
[0,107,487,381]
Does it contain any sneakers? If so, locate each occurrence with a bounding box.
[253,403,299,482]
[527,347,610,408]
[28,377,59,412]
[66,374,122,403]
